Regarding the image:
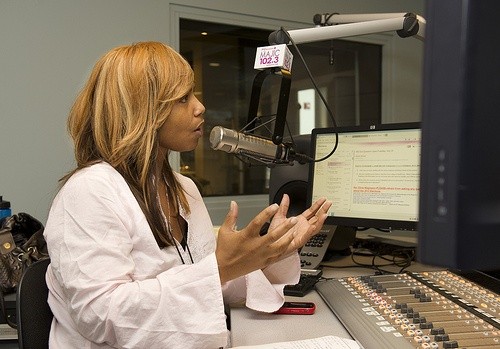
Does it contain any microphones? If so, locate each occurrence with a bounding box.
[210,125,313,163]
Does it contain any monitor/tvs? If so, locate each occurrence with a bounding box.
[305,122,422,255]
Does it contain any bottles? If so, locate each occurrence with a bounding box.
[0,194,11,223]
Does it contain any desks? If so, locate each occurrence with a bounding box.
[228,252,444,348]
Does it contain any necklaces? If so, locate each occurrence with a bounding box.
[156,179,172,235]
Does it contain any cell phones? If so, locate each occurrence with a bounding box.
[271,302,316,315]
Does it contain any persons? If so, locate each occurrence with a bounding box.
[41,41,332,349]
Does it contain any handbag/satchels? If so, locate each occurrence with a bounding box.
[0,212,50,329]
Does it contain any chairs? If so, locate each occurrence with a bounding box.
[15,257,54,349]
